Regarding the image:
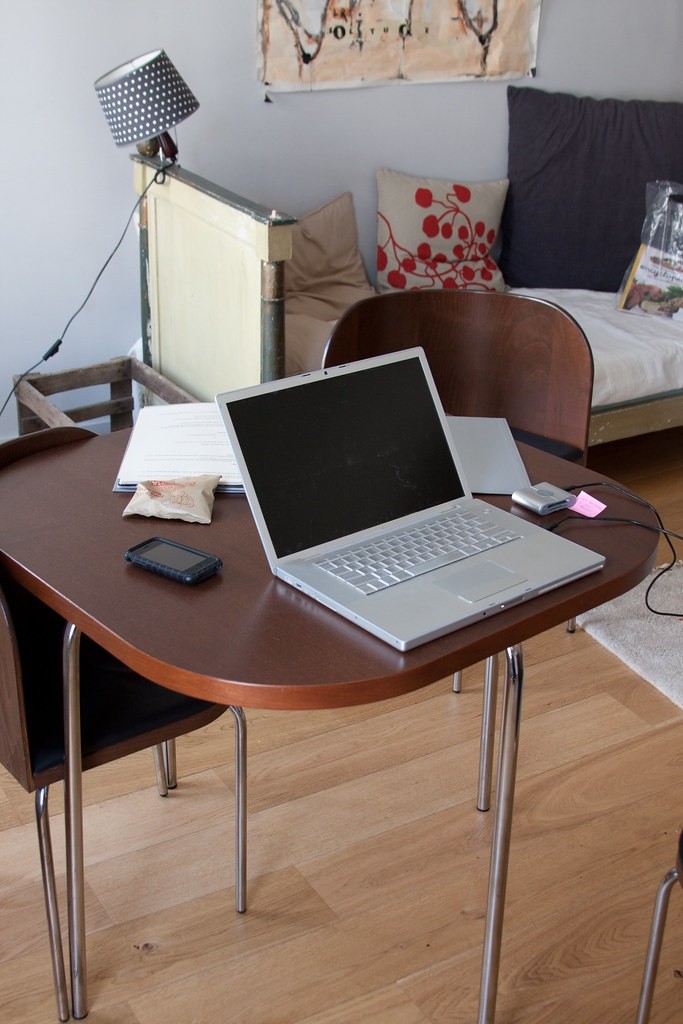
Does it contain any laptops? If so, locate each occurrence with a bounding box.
[215,347,608,652]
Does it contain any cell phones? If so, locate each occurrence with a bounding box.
[124,536,223,587]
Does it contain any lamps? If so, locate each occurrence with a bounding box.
[94,49,200,163]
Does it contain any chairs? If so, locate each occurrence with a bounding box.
[1,579,251,1021]
[321,289,595,694]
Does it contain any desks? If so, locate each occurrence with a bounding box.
[1,403,662,1024]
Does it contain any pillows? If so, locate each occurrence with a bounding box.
[376,167,511,293]
[499,86,683,293]
[284,192,383,322]
